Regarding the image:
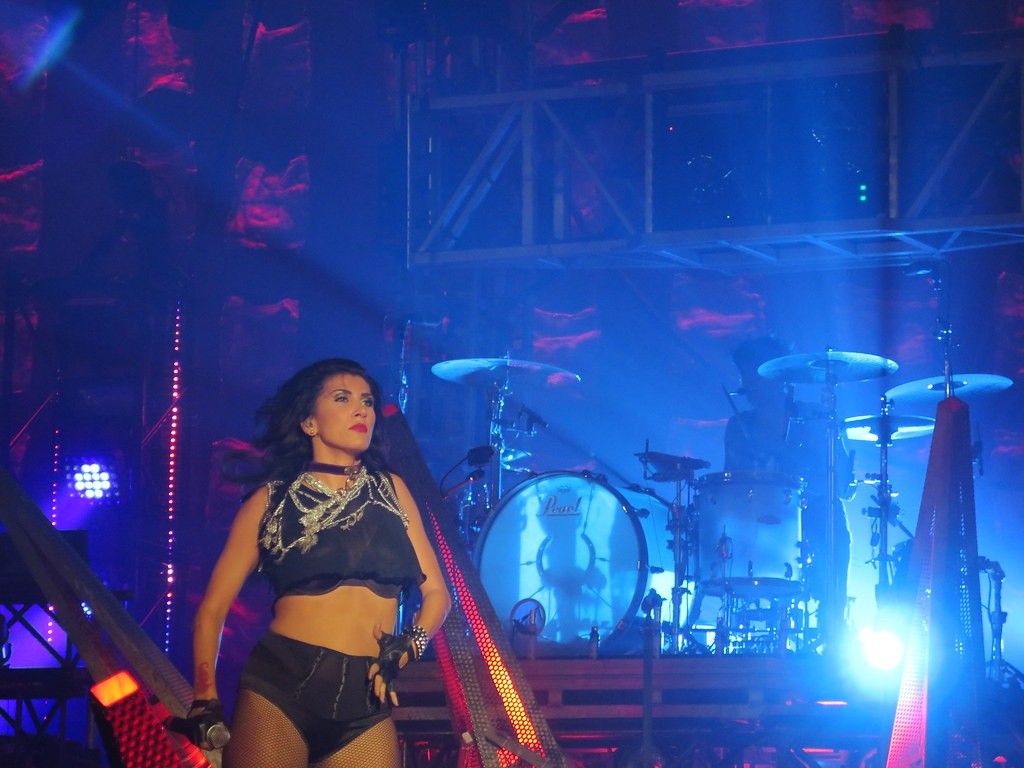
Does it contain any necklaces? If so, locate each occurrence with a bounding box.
[262,461,410,561]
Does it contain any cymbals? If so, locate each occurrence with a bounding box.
[884,373,1012,412]
[843,414,936,441]
[430,351,581,390]
[757,345,899,384]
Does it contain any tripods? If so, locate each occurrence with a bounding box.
[625,480,713,658]
[984,569,1024,691]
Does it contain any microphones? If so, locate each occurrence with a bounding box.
[164,715,230,749]
[975,422,984,477]
[870,494,914,539]
[496,419,535,436]
[783,385,795,440]
[653,469,694,482]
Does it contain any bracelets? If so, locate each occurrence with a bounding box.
[400,624,429,663]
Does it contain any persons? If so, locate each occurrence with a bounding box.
[721,338,858,657]
[186,357,453,768]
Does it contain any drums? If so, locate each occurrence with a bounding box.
[695,472,807,598]
[467,470,650,657]
